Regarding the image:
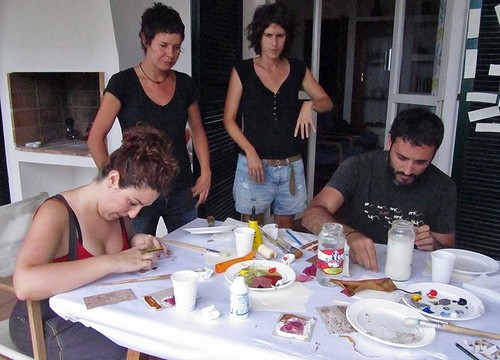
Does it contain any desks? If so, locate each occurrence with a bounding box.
[49,218,500,360]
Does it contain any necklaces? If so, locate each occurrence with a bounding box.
[139,62,169,83]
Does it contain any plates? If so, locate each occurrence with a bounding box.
[182,225,236,235]
[345,298,436,348]
[402,282,485,321]
[438,248,500,276]
[224,259,297,291]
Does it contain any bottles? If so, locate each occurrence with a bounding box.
[230,276,249,320]
[384,220,415,282]
[315,222,345,288]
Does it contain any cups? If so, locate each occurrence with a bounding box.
[430,250,456,285]
[170,269,198,311]
[234,227,255,257]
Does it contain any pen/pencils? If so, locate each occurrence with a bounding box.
[142,247,164,254]
[286,230,302,245]
[456,343,479,360]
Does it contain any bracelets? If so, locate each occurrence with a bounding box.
[345,230,359,237]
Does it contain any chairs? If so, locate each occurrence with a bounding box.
[314,134,353,197]
[0,192,48,360]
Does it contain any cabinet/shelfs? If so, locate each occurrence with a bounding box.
[343,16,438,150]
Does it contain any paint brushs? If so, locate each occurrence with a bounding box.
[260,229,290,254]
[404,318,500,339]
[171,241,229,256]
[96,270,205,286]
[278,238,302,258]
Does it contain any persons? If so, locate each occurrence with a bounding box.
[222,0,332,230]
[301,108,458,273]
[9,122,180,360]
[88,1,211,236]
